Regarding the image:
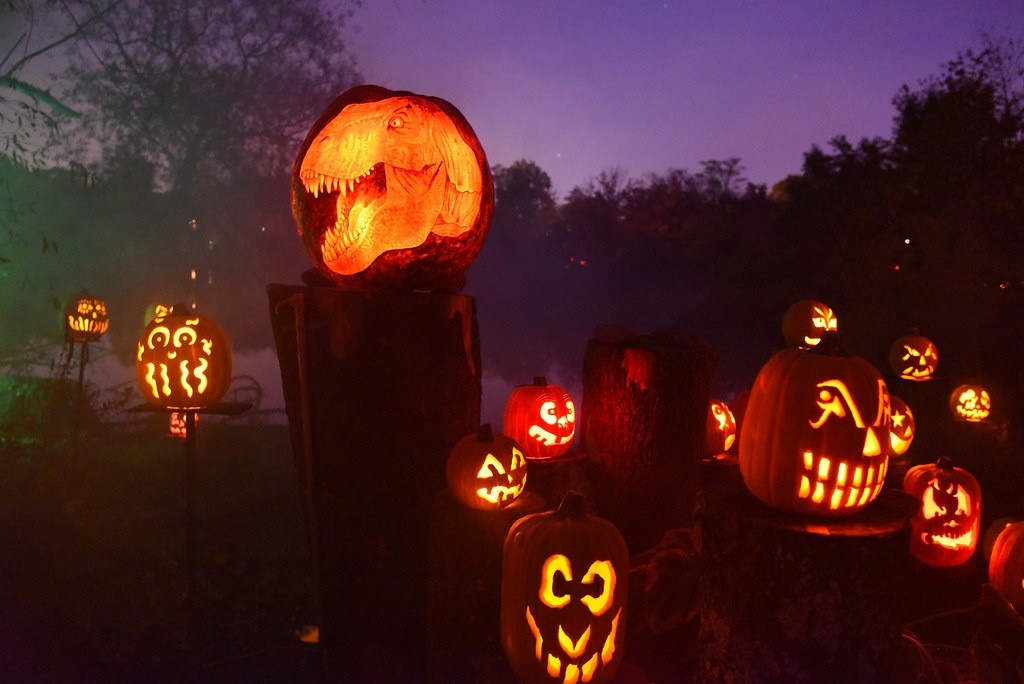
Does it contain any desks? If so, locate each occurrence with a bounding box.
[125,402,254,609]
